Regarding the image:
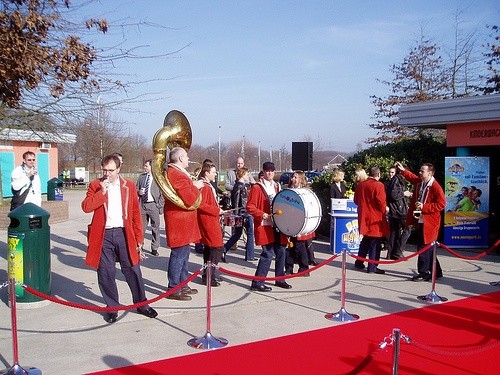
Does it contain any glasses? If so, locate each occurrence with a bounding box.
[26,158,35,161]
[100,166,119,174]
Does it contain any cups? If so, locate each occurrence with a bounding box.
[105,175,111,184]
[33,167,37,174]
[141,188,145,194]
[406,187,411,191]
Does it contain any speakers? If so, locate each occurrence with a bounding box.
[292,142,313,172]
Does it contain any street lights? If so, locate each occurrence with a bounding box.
[259,140,262,172]
[242,134,244,158]
[97,95,104,176]
[270,145,273,162]
[279,148,282,171]
[218,124,221,172]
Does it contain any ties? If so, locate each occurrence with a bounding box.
[142,175,150,203]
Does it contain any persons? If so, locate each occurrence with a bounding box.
[113,152,123,262]
[246,162,292,292]
[329,169,347,199]
[225,155,256,250]
[386,163,413,261]
[81,154,158,323]
[221,168,258,263]
[394,161,447,282]
[197,164,225,287]
[306,238,320,266]
[354,165,391,274]
[194,167,201,177]
[163,147,205,301]
[447,185,482,212]
[63,167,70,187]
[194,159,227,253]
[351,168,368,192]
[285,171,316,277]
[135,159,165,256]
[259,171,264,181]
[9,150,42,211]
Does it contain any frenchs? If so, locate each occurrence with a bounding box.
[151,110,202,210]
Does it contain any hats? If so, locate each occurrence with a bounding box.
[263,162,274,170]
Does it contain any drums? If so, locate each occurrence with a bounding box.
[223,213,243,227]
[271,188,322,237]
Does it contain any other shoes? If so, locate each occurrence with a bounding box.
[308,259,319,266]
[429,272,442,279]
[168,293,193,300]
[285,271,293,275]
[195,249,203,255]
[181,286,198,294]
[413,271,430,281]
[253,258,258,260]
[390,254,406,261]
[152,249,159,256]
[202,279,220,287]
[355,263,368,272]
[221,253,226,262]
[367,268,385,274]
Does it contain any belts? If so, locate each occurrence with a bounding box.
[144,201,155,204]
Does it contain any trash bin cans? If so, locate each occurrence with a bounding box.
[47,177,64,202]
[7,202,51,309]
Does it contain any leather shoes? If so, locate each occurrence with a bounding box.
[137,306,157,318]
[251,284,272,292]
[276,281,291,288]
[105,311,117,323]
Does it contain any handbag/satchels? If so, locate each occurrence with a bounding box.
[10,189,28,210]
